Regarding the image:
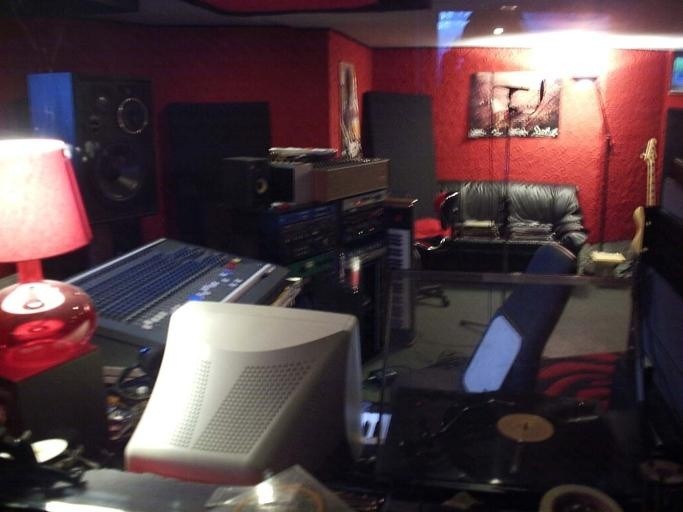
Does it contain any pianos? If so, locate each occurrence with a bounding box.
[386,217,413,336]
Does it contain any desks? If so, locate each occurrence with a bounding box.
[103,275,309,433]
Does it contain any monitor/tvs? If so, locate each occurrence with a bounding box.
[667,49,683,96]
[124,300,365,487]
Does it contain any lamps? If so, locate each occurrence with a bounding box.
[1,136,98,344]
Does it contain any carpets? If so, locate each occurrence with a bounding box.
[534,351,625,407]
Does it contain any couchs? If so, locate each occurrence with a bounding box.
[436,179,588,275]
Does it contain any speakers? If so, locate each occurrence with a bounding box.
[27,74,162,225]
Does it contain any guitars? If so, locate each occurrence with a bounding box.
[631,138,659,256]
